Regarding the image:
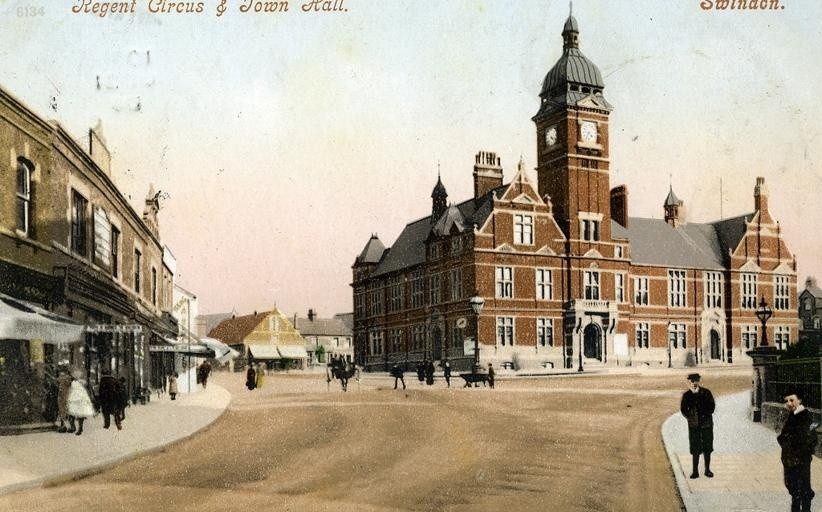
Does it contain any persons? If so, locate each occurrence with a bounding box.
[681,374,715,478]
[247,364,256,390]
[327,353,346,378]
[169,371,177,400]
[255,364,265,389]
[390,360,494,389]
[777,391,818,512]
[41,364,128,436]
[200,360,212,388]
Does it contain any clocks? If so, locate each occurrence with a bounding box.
[545,128,557,145]
[581,122,597,143]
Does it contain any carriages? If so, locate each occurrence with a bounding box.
[323,351,362,394]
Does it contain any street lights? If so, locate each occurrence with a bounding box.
[753,291,774,348]
[185,294,198,394]
[470,292,486,376]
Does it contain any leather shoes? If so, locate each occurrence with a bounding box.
[705,470,713,477]
[690,473,699,478]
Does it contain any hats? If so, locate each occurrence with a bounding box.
[688,374,701,380]
